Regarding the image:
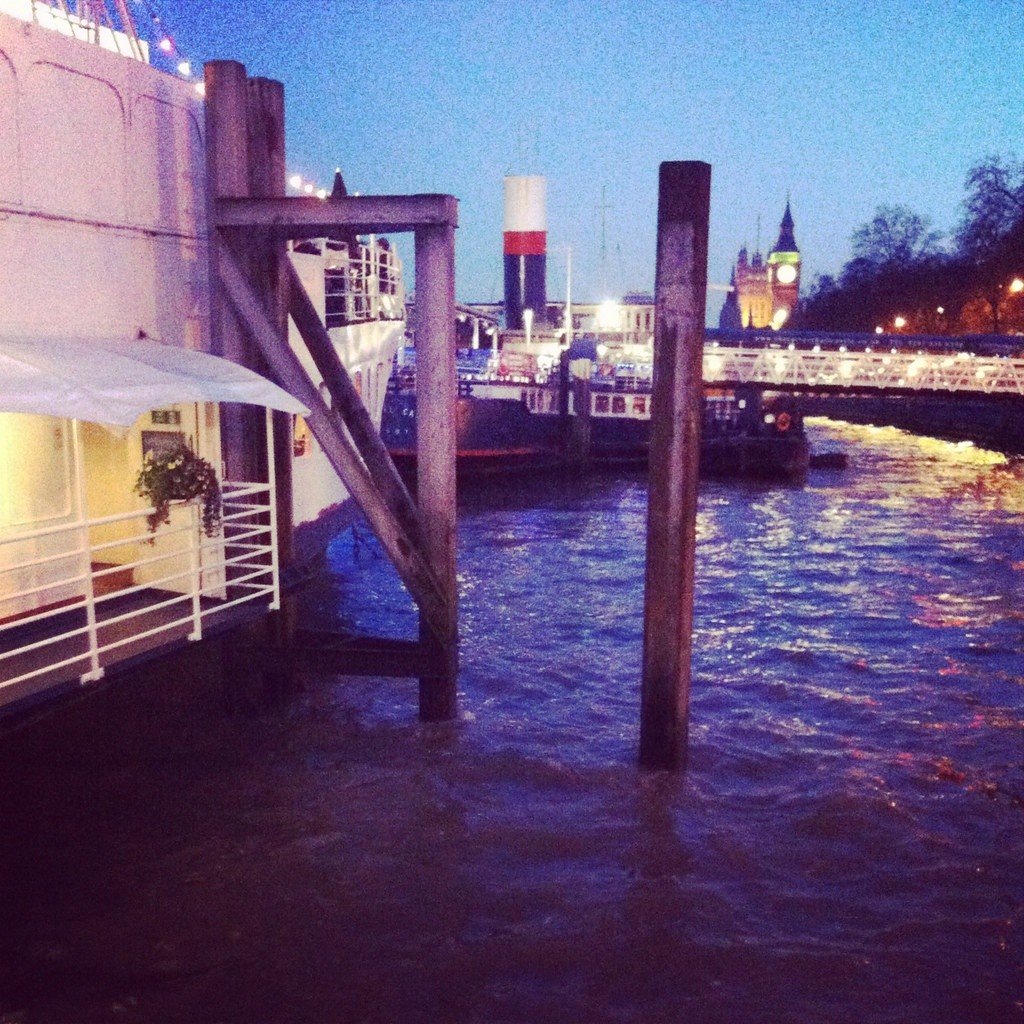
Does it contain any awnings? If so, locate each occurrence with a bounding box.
[0,333,312,679]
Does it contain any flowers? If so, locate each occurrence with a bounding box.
[132,442,223,547]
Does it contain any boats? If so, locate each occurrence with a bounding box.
[0,1,846,754]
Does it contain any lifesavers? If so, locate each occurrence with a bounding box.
[498,367,508,375]
[777,413,790,431]
